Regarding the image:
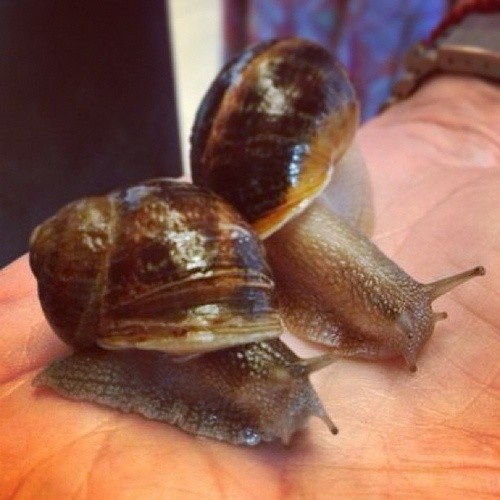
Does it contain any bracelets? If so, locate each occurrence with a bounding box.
[371,9,500,121]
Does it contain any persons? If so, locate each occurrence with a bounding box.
[1,0,500,500]
